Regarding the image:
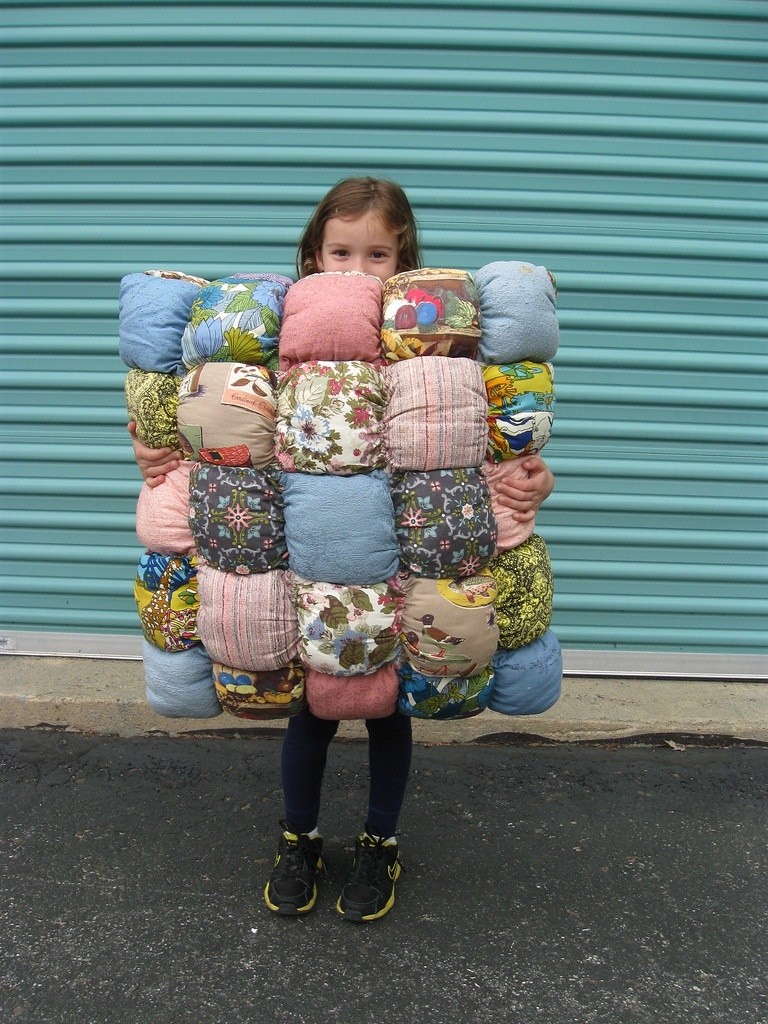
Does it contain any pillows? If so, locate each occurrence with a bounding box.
[118,260,562,721]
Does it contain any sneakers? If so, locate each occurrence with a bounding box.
[264,819,328,915]
[337,832,407,921]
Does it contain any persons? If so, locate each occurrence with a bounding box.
[128,175,558,920]
